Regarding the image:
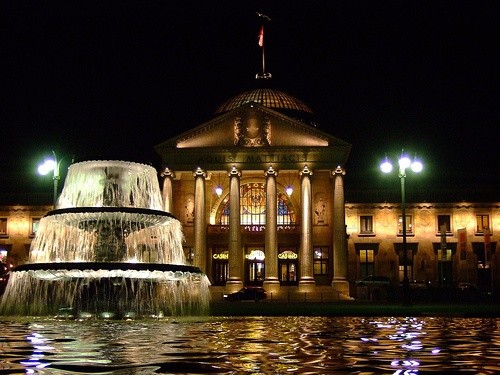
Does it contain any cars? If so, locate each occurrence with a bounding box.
[458,281,478,290]
[356,274,391,286]
[223,287,266,301]
[402,279,432,288]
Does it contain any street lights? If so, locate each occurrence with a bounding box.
[38,148,76,210]
[380,147,424,286]
